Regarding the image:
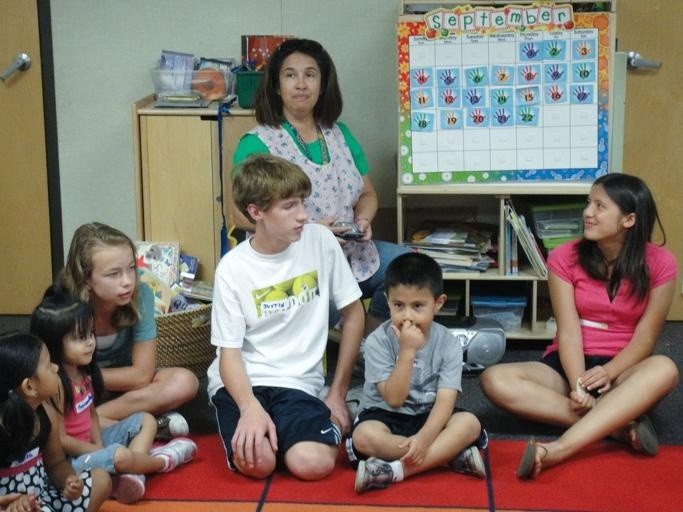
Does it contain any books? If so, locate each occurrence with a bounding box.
[179,255,198,292]
[404,199,578,279]
[132,240,180,293]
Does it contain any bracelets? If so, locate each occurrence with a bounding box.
[354,218,369,224]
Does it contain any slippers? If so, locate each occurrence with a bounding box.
[615,412,660,456]
[516,432,549,481]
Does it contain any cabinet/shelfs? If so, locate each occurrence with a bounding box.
[130,99,259,303]
[395,183,595,342]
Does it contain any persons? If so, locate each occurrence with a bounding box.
[479,172,681,481]
[204,152,366,482]
[0,329,114,512]
[232,38,418,329]
[29,283,199,504]
[55,222,201,444]
[344,252,489,494]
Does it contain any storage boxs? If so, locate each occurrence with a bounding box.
[529,201,590,239]
[544,235,580,253]
[469,294,529,330]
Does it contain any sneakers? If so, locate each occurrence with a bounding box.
[354,455,394,494]
[154,411,190,444]
[148,436,199,473]
[448,445,487,479]
[109,473,147,505]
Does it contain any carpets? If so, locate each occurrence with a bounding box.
[105,431,682,511]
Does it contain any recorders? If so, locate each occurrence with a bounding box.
[433,315,506,372]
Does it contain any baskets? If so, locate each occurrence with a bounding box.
[155,303,214,381]
